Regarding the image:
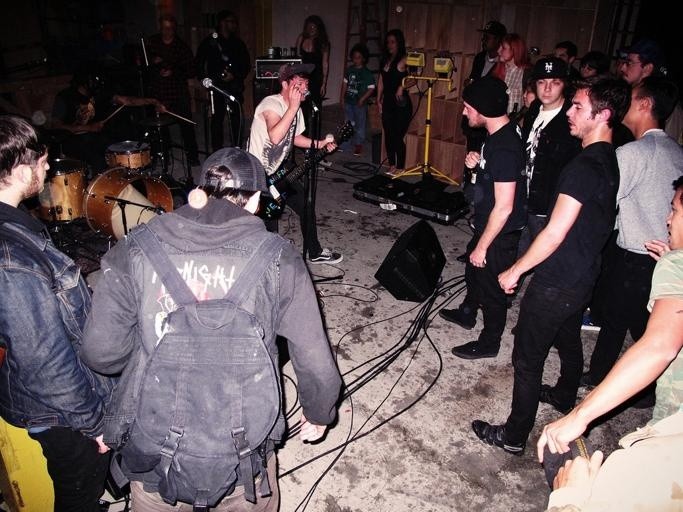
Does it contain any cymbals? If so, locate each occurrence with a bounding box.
[139,112,174,127]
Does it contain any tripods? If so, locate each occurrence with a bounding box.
[302,115,344,295]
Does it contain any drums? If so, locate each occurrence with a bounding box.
[39,168,85,224]
[84,167,173,241]
[105,139,152,170]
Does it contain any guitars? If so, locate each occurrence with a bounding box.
[259,120,355,219]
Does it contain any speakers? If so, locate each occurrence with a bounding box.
[372,217,448,302]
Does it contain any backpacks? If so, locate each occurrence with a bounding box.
[117,221,287,512]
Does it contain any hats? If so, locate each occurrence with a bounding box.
[531,57,567,81]
[461,79,510,118]
[476,20,507,36]
[199,146,268,192]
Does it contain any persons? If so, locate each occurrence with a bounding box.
[0,115,120,512]
[579,76,683,408]
[295,14,331,140]
[36,87,93,178]
[516,59,572,260]
[439,75,527,360]
[536,174,683,487]
[554,41,579,79]
[512,67,535,116]
[580,50,607,78]
[377,29,413,177]
[497,34,528,115]
[138,13,196,169]
[194,10,251,149]
[340,44,376,156]
[621,38,683,149]
[246,63,338,261]
[472,72,631,457]
[460,20,507,189]
[80,146,342,512]
[616,45,627,80]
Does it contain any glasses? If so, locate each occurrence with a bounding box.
[622,60,640,66]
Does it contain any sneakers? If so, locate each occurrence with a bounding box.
[452,340,498,360]
[581,373,599,387]
[471,419,526,457]
[310,248,343,265]
[438,308,476,330]
[540,383,575,416]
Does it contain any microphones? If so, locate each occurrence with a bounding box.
[201,77,239,105]
[303,89,320,113]
